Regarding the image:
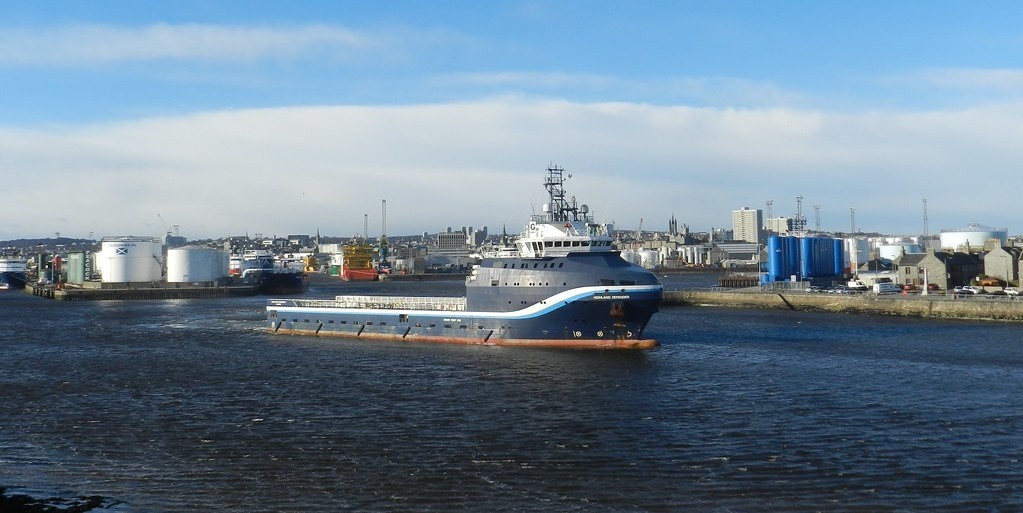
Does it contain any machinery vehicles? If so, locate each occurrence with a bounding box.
[682,259,695,268]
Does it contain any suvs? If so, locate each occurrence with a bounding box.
[1003,287,1020,296]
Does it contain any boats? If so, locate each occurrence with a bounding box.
[338,237,377,281]
[270,268,309,295]
[265,165,664,350]
[0,279,10,290]
[285,255,330,279]
[326,251,342,277]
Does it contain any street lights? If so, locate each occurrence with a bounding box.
[921,268,927,295]
[52,251,55,286]
[759,243,762,293]
[875,258,878,283]
[856,251,861,274]
[799,259,804,292]
[892,255,897,283]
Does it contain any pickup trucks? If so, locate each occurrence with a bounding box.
[379,268,392,274]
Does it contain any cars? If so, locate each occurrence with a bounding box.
[962,286,982,294]
[905,284,916,290]
[928,284,939,290]
[954,286,962,293]
[835,285,846,290]
[805,285,863,295]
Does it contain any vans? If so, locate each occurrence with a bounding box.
[873,282,901,294]
[847,281,867,290]
